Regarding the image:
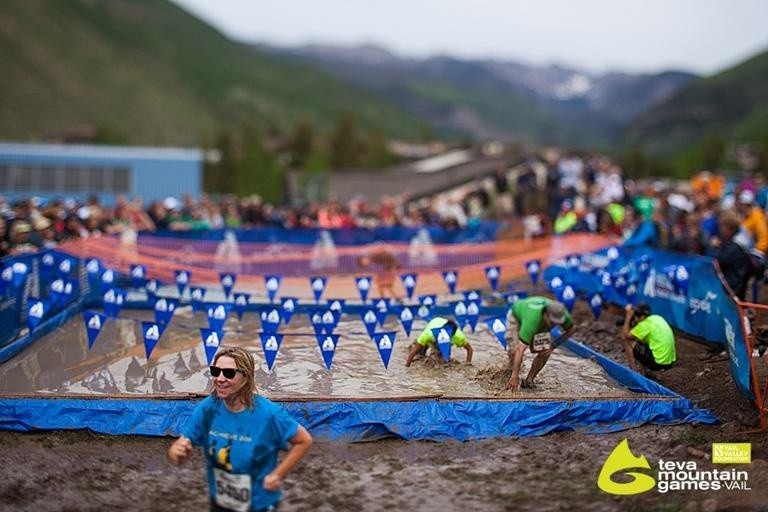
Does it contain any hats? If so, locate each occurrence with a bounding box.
[547,302,564,324]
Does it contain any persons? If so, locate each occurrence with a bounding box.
[167,346,313,512]
[621,301,677,375]
[405,316,474,367]
[0,134,768,303]
[505,295,580,393]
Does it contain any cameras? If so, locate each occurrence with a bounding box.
[616,316,637,328]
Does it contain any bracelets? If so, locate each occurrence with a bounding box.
[465,362,473,365]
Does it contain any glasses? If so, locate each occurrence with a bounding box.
[209,366,244,378]
